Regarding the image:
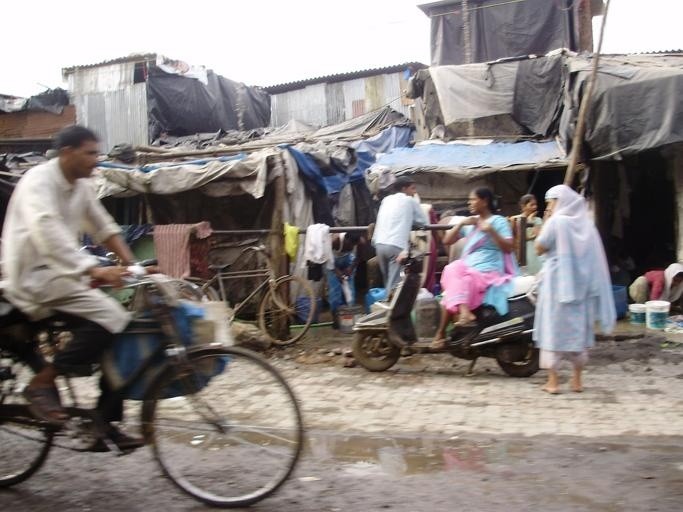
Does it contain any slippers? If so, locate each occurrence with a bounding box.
[433,337,446,348]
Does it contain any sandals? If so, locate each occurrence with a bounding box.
[73,421,142,452]
[23,386,69,423]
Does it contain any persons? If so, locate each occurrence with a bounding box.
[372,176,430,303]
[510,191,542,226]
[326,228,361,328]
[436,207,474,257]
[413,192,449,296]
[425,185,517,348]
[0,125,163,427]
[528,183,620,395]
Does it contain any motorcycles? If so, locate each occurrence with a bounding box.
[352,241,539,378]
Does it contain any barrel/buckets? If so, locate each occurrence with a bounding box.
[338,304,362,334]
[612,286,627,319]
[645,300,670,330]
[297,296,323,323]
[366,288,386,312]
[629,304,644,325]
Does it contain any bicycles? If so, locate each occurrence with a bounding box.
[0,258,305,509]
[179,236,316,348]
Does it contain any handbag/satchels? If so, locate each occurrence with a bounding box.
[102,299,229,400]
